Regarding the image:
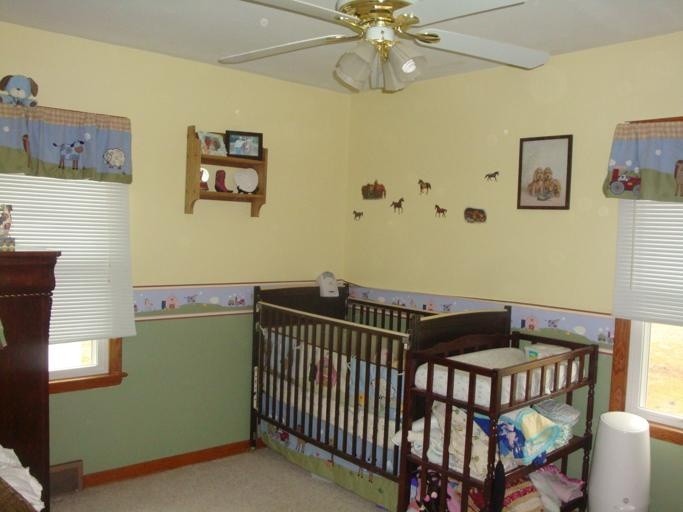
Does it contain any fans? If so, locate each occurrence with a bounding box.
[216,0,549,92]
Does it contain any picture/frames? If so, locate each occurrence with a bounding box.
[49,460,84,495]
[517,134,573,209]
[226,131,263,160]
[198,132,228,157]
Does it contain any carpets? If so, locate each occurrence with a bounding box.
[51,448,384,511]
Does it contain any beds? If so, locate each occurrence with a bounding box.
[249,283,511,512]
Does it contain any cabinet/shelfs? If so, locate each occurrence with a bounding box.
[185,125,269,218]
[0,251,62,512]
[396,331,599,512]
